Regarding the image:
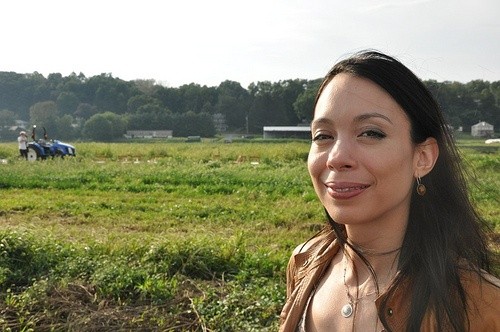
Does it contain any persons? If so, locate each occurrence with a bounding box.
[17,131,28,160]
[277,48,499,332]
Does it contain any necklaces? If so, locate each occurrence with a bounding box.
[341,237,390,319]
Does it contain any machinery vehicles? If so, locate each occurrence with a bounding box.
[25,125,76,162]
